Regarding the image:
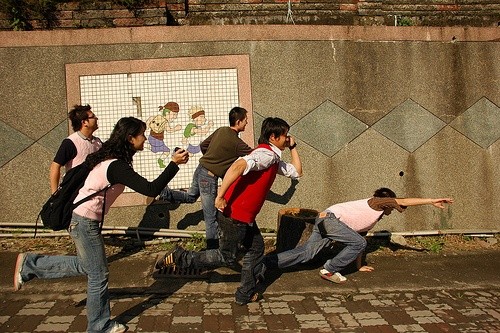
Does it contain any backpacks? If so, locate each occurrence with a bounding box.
[39,152,128,231]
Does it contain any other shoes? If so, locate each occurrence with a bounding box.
[154,185,162,200]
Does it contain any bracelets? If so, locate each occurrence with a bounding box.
[289,143,296,150]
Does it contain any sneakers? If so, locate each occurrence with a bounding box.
[236,292,259,306]
[319,269,347,283]
[155,244,182,269]
[87,320,127,333]
[13,252,26,291]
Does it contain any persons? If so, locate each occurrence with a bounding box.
[156,107,254,250]
[254,188,454,284]
[14,116,189,333]
[50,104,103,195]
[156,118,302,304]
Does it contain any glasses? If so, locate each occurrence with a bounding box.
[86,115,96,119]
